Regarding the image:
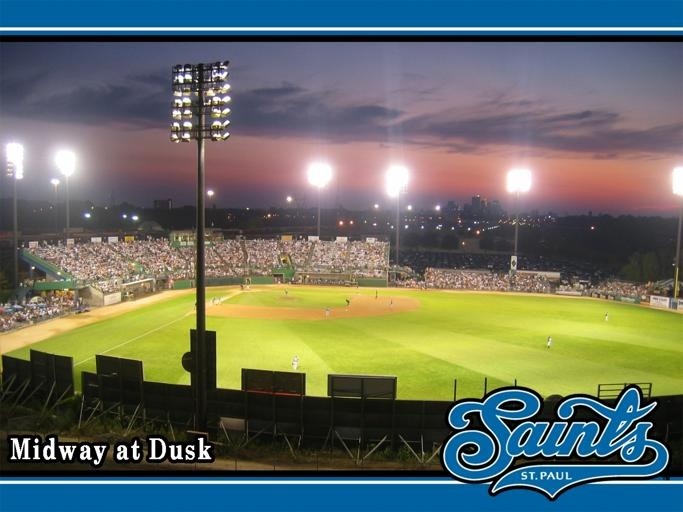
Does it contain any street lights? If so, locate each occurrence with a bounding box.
[384,164,410,265]
[670,165,683,300]
[307,160,334,240]
[507,167,533,271]
[169,58,233,446]
[4,141,26,289]
[52,179,58,214]
[55,149,77,227]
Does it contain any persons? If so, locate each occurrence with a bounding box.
[604,312,608,321]
[291,356,299,370]
[345,299,349,310]
[204,237,387,305]
[546,336,551,349]
[324,307,331,319]
[0,236,196,331]
[388,263,670,307]
